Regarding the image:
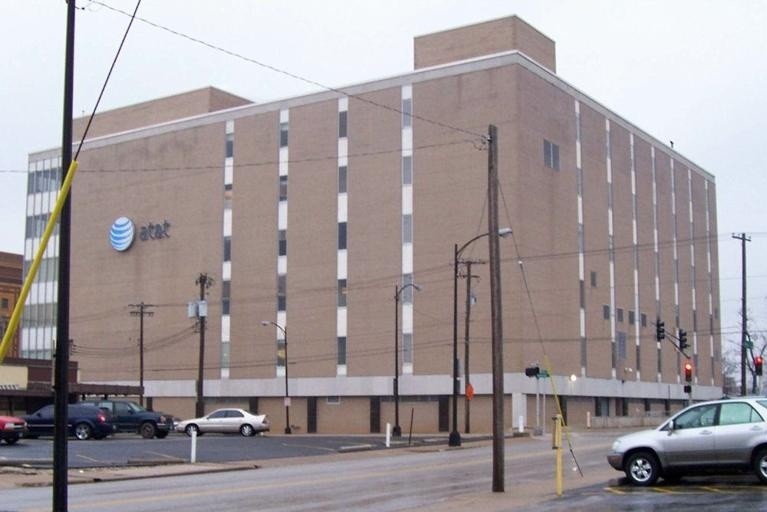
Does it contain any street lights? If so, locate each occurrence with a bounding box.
[447,228,512,447]
[261,319,290,434]
[394,281,419,436]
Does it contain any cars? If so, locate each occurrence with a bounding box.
[1,398,269,443]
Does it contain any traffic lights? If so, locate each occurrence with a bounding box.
[678,329,687,349]
[755,357,762,376]
[685,364,691,380]
[684,385,690,393]
[655,318,664,340]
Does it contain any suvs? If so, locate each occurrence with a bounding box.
[605,395,766,489]
